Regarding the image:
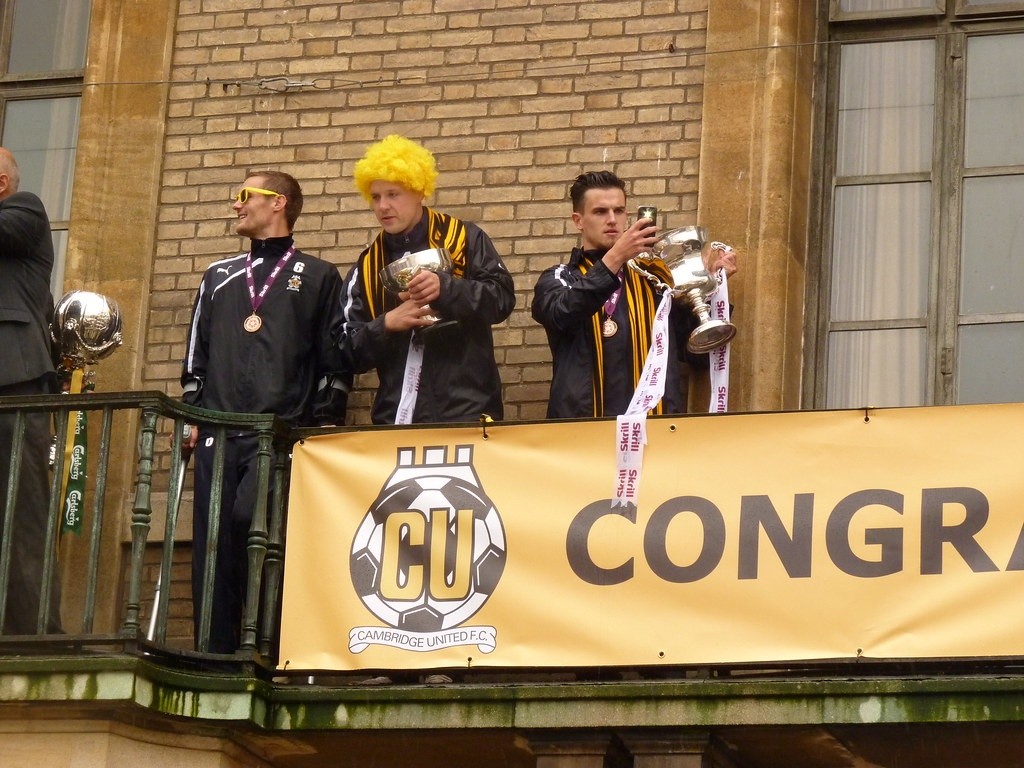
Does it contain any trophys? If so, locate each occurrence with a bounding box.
[379,247,459,335]
[628,226,737,353]
[49,290,123,374]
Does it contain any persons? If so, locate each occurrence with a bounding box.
[530,170,738,419]
[337,134,516,425]
[170,170,351,659]
[0,148,60,644]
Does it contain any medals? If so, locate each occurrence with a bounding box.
[603,318,617,337]
[244,312,262,332]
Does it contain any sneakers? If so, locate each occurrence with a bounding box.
[426,673,452,683]
[363,676,392,685]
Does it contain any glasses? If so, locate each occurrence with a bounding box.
[236,187,278,204]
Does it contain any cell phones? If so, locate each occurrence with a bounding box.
[638,207,658,247]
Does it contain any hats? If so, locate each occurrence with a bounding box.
[354,134,438,201]
